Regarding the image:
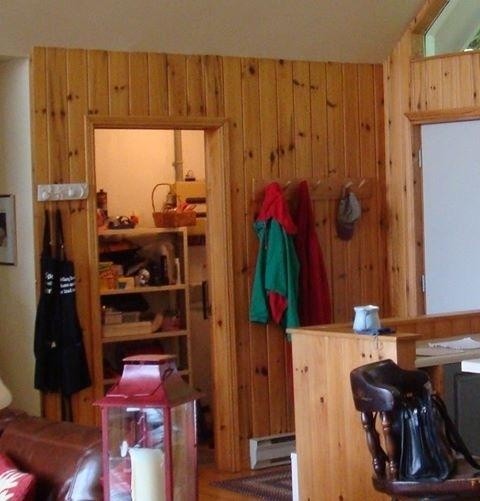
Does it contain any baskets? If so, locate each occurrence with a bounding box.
[151,184,197,228]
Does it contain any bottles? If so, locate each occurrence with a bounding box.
[96,188,108,216]
[158,254,170,285]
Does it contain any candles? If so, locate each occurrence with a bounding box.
[128,445,165,501]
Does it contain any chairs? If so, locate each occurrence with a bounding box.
[348,359,480,501]
[0,406,107,501]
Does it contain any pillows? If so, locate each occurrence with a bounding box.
[0,449,38,501]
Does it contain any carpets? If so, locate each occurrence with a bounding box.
[208,466,293,501]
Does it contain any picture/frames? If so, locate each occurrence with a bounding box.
[0,191,20,270]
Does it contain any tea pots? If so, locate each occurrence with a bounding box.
[352,303,382,331]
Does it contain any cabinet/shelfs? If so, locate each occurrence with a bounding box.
[96,225,200,398]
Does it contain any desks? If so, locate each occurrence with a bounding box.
[459,355,480,379]
[283,307,480,501]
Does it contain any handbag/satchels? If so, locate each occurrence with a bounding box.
[389,379,480,482]
